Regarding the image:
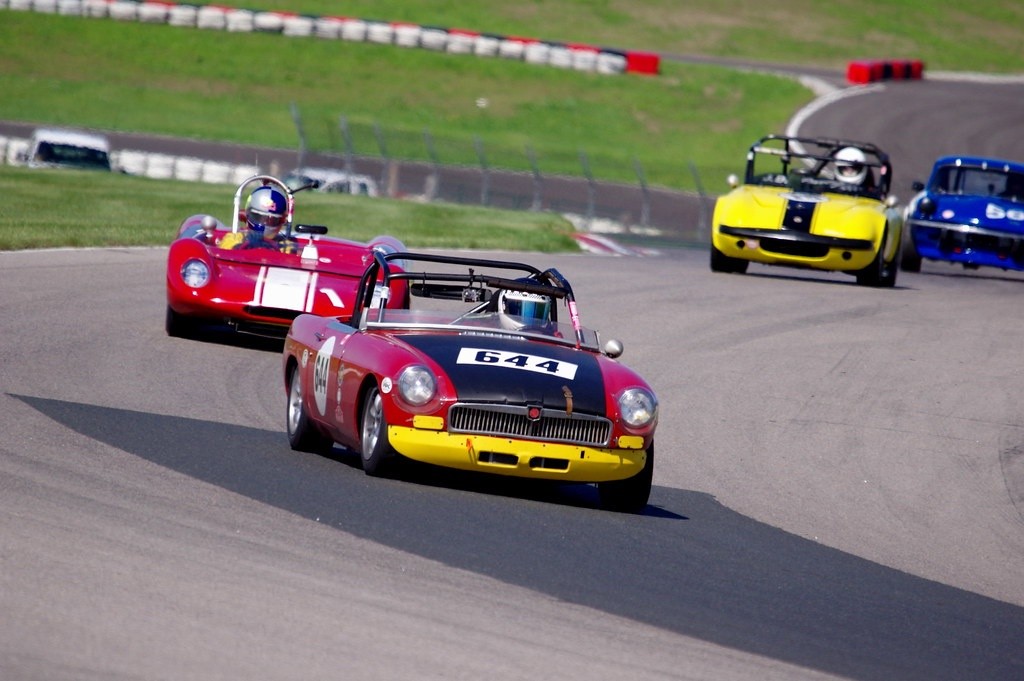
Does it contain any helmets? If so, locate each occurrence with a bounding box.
[498,277,553,332]
[244,186,287,240]
[832,146,868,185]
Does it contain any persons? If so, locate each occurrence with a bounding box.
[833,147,867,185]
[498,278,564,338]
[219,188,296,254]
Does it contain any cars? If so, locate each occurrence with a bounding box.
[14,129,118,176]
[283,253,660,509]
[286,167,377,197]
[903,155,1024,273]
[166,173,411,341]
[710,134,903,288]
